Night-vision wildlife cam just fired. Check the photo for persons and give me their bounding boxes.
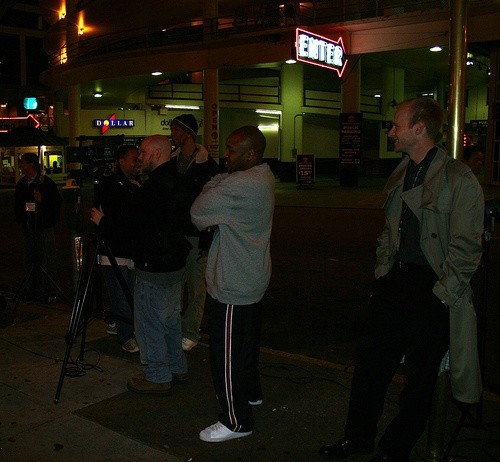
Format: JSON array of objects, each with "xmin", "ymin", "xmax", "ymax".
[
  {"xmin": 170, "ymin": 114, "xmax": 220, "ymax": 350},
  {"xmin": 90, "ymin": 135, "xmax": 189, "ymax": 393},
  {"xmin": 95, "ymin": 145, "xmax": 146, "ymax": 352},
  {"xmin": 189, "ymin": 126, "xmax": 276, "ymax": 443},
  {"xmin": 319, "ymin": 97, "xmax": 485, "ymax": 462},
  {"xmin": 14, "ymin": 152, "xmax": 60, "ymax": 304}
]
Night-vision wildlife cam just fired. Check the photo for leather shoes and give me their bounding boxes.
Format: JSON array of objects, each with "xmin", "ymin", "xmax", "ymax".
[
  {"xmin": 371, "ymin": 452, "xmax": 393, "ymax": 462},
  {"xmin": 321, "ymin": 434, "xmax": 374, "ymax": 457}
]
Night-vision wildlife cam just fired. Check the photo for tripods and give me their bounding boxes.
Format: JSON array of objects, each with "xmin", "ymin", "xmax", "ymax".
[
  {"xmin": 443, "ymin": 230, "xmax": 500, "ymax": 462},
  {"xmin": 8, "ymin": 176, "xmax": 76, "ymax": 326},
  {"xmin": 54, "ymin": 161, "xmax": 134, "ymax": 404}
]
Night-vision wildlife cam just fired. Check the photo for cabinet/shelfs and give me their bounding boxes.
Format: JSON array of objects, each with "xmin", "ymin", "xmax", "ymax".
[{"xmin": 64, "ymin": 134, "xmax": 173, "ymax": 181}]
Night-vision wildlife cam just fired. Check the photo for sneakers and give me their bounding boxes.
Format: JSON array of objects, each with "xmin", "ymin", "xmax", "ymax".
[
  {"xmin": 199, "ymin": 421, "xmax": 253, "ymax": 443},
  {"xmin": 172, "ymin": 373, "xmax": 188, "ymax": 381},
  {"xmin": 127, "ymin": 378, "xmax": 171, "ymax": 394}
]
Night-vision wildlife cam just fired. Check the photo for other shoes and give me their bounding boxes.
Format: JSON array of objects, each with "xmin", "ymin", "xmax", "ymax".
[
  {"xmin": 122, "ymin": 338, "xmax": 139, "ymax": 352},
  {"xmin": 181, "ymin": 337, "xmax": 197, "ymax": 350},
  {"xmin": 107, "ymin": 324, "xmax": 117, "ymax": 334}
]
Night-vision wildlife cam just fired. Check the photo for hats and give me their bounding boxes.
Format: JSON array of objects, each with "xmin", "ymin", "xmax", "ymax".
[{"xmin": 173, "ymin": 113, "xmax": 199, "ymax": 139}]
